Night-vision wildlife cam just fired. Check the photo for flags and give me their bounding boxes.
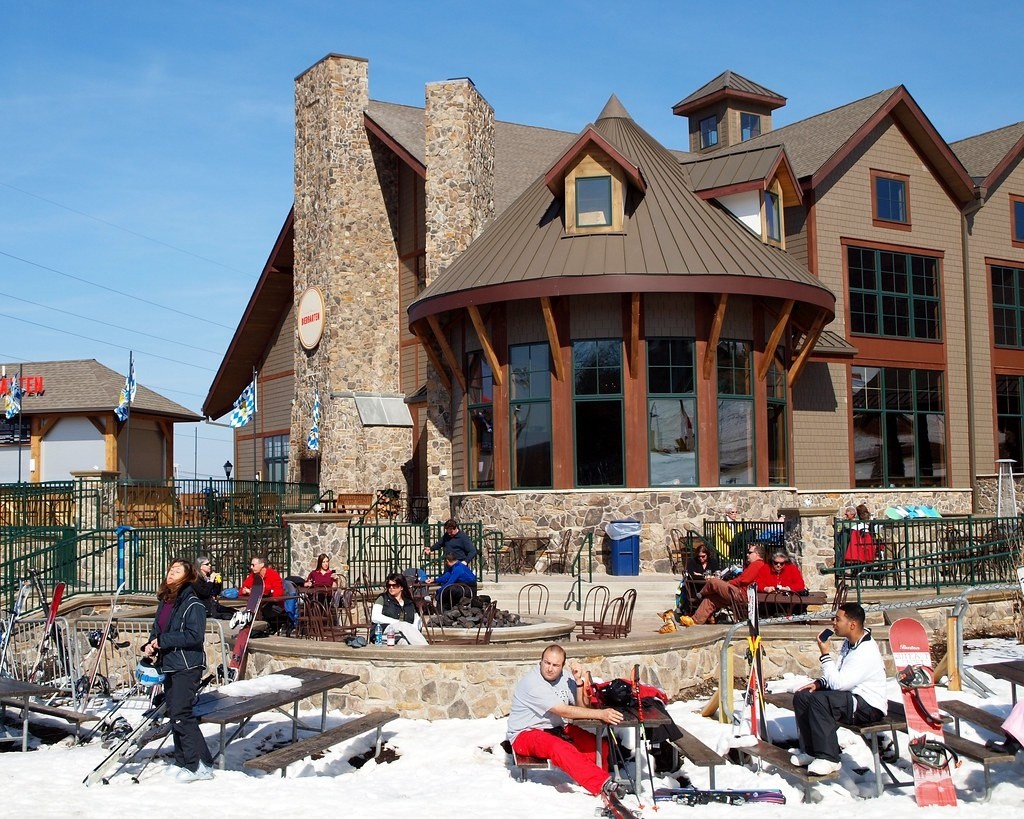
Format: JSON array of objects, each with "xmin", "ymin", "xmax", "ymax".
[
  {"xmin": 114, "ymin": 357, "xmax": 137, "ymax": 422},
  {"xmin": 306, "ymin": 389, "xmax": 322, "ymax": 452},
  {"xmin": 1, "ymin": 370, "xmax": 27, "ymax": 419},
  {"xmin": 228, "ymin": 380, "xmax": 257, "ymax": 429}
]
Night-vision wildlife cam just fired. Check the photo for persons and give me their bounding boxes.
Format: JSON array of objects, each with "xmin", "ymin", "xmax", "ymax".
[
  {"xmin": 238, "ymin": 556, "xmax": 286, "ymax": 635},
  {"xmin": 368, "ymin": 572, "xmax": 429, "ymax": 645},
  {"xmin": 191, "ymin": 554, "xmax": 233, "ymax": 620},
  {"xmin": 789, "ymin": 603, "xmax": 890, "ymax": 777},
  {"xmin": 141, "ymin": 557, "xmax": 216, "ymax": 782},
  {"xmin": 424, "ymin": 520, "xmax": 479, "ymax": 574},
  {"xmin": 425, "ymin": 552, "xmax": 478, "ymax": 615},
  {"xmin": 506, "ymin": 645, "xmax": 629, "ymax": 800},
  {"xmin": 303, "ymin": 554, "xmax": 340, "ymax": 614},
  {"xmin": 986, "ymin": 695, "xmax": 1023, "ymax": 756},
  {"xmin": 663, "ymin": 505, "xmax": 885, "ymax": 626}
]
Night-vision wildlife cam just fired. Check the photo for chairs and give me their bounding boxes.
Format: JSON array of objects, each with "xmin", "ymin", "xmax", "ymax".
[{"xmin": 212, "ymin": 517, "xmax": 1024, "ymax": 644}]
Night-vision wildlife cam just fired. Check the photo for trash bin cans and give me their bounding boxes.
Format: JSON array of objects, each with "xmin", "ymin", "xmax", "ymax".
[{"xmin": 604, "ymin": 519, "xmax": 642, "ymax": 576}]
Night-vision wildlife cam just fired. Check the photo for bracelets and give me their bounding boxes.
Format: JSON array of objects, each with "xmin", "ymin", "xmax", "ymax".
[{"xmin": 575, "ymin": 680, "xmax": 585, "ymax": 688}]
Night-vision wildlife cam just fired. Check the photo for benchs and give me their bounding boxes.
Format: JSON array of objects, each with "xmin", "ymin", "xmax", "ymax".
[
  {"xmin": 143, "ymin": 715, "xmax": 246, "ymax": 748},
  {"xmin": 330, "ymin": 494, "xmax": 373, "ymax": 526},
  {"xmin": 938, "ymin": 700, "xmax": 1007, "ymax": 739},
  {"xmin": 736, "ymin": 735, "xmax": 841, "ymax": 804},
  {"xmin": 511, "ymin": 745, "xmax": 549, "ymax": 782},
  {"xmin": 242, "ymin": 711, "xmax": 401, "ymax": 778},
  {"xmin": 0, "ymin": 508, "xmax": 167, "ymax": 527},
  {"xmin": 895, "ymin": 727, "xmax": 1015, "ymax": 802},
  {"xmin": 672, "ymin": 723, "xmax": 726, "ymax": 790},
  {"xmin": 0, "ymin": 696, "xmax": 102, "ymax": 747}
]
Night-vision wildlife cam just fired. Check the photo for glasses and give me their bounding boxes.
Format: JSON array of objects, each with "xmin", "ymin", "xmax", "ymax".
[
  {"xmin": 773, "ymin": 560, "xmax": 786, "ymax": 566},
  {"xmin": 250, "ymin": 563, "xmax": 262, "ymax": 567},
  {"xmin": 728, "ymin": 510, "xmax": 738, "ymax": 514},
  {"xmin": 386, "ymin": 583, "xmax": 404, "ymax": 589},
  {"xmin": 748, "ymin": 551, "xmax": 757, "ymax": 555},
  {"xmin": 201, "ymin": 562, "xmax": 211, "ymax": 567},
  {"xmin": 697, "ymin": 554, "xmax": 708, "ymax": 558}
]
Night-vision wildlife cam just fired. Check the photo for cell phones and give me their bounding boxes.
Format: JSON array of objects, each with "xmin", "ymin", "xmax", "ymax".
[
  {"xmin": 819, "ymin": 629, "xmax": 834, "ymax": 642},
  {"xmin": 332, "ymin": 570, "xmax": 336, "ymax": 573}
]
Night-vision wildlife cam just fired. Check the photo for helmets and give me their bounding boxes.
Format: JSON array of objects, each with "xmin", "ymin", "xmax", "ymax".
[{"xmin": 135, "ymin": 658, "xmax": 168, "ymax": 689}]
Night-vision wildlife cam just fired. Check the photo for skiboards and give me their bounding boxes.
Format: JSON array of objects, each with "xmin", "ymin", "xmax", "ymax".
[
  {"xmin": 18, "ymin": 581, "xmax": 67, "ymax": 719},
  {"xmin": 747, "ymin": 581, "xmax": 771, "ymax": 742},
  {"xmin": 0, "ymin": 584, "xmax": 28, "ymax": 677},
  {"xmin": 80, "ymin": 582, "xmax": 126, "ymax": 714}
]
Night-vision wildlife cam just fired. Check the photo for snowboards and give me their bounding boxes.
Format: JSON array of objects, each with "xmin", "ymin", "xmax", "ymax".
[
  {"xmin": 226, "ymin": 572, "xmax": 264, "ymax": 686},
  {"xmin": 651, "ymin": 787, "xmax": 787, "ymax": 806},
  {"xmin": 601, "ymin": 790, "xmax": 645, "ymax": 819},
  {"xmin": 888, "ymin": 618, "xmax": 962, "ymax": 807}
]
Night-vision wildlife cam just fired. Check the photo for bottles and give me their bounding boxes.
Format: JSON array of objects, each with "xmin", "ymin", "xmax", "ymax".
[
  {"xmin": 703, "ymin": 568, "xmax": 721, "ymax": 580},
  {"xmin": 387, "ymin": 625, "xmax": 395, "ymax": 646},
  {"xmin": 374, "ymin": 621, "xmax": 382, "ymax": 647}
]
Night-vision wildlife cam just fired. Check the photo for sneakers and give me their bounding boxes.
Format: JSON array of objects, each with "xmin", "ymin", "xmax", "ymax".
[
  {"xmin": 679, "ymin": 614, "xmax": 698, "ymax": 627},
  {"xmin": 607, "ymin": 780, "xmax": 629, "ymax": 800},
  {"xmin": 807, "ymin": 758, "xmax": 842, "ymax": 775},
  {"xmin": 790, "ymin": 751, "xmax": 817, "ymax": 767},
  {"xmin": 169, "ymin": 764, "xmax": 189, "ymax": 776},
  {"xmin": 176, "ymin": 756, "xmax": 214, "ymax": 782}
]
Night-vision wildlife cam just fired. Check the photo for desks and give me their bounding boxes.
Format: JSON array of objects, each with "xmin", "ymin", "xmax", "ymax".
[
  {"xmin": 764, "ymin": 690, "xmax": 954, "ymax": 798},
  {"xmin": 168, "ymin": 667, "xmax": 360, "ymax": 772},
  {"xmin": 0, "ymin": 678, "xmax": 61, "ymax": 754},
  {"xmin": 490, "ymin": 536, "xmax": 552, "ymax": 575},
  {"xmin": 573, "ymin": 705, "xmax": 671, "ymax": 797},
  {"xmin": 974, "ymin": 660, "xmax": 1024, "ymax": 708},
  {"xmin": 314, "ymin": 499, "xmax": 339, "ymax": 513}
]
